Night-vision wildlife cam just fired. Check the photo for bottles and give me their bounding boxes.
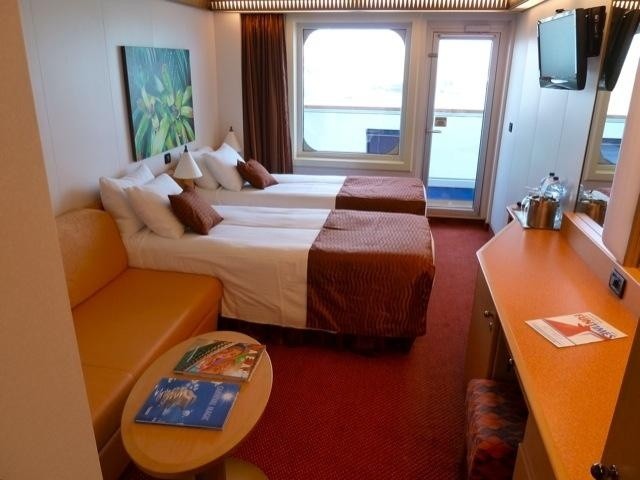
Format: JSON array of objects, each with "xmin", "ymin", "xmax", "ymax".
[
  {"xmin": 543, "ymin": 177, "xmax": 564, "ymax": 230},
  {"xmin": 538, "ymin": 172, "xmax": 556, "ymax": 199}
]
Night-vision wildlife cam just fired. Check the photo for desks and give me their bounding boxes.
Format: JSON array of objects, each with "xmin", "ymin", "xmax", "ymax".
[{"xmin": 467, "ymin": 203, "xmax": 637, "ymax": 477}]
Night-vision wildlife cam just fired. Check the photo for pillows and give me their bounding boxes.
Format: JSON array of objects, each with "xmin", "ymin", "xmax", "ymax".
[
  {"xmin": 203, "ymin": 142, "xmax": 246, "ymax": 192},
  {"xmin": 100, "ymin": 163, "xmax": 155, "ymax": 240},
  {"xmin": 236, "ymin": 159, "xmax": 279, "ymax": 189},
  {"xmin": 167, "ymin": 186, "xmax": 224, "ymax": 235},
  {"xmin": 123, "ymin": 173, "xmax": 186, "ymax": 241},
  {"xmin": 176, "ymin": 146, "xmax": 220, "ymax": 190}
]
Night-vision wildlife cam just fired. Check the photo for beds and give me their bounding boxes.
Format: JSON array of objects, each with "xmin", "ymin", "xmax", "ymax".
[
  {"xmin": 99, "ymin": 204, "xmax": 433, "ymax": 352},
  {"xmin": 173, "ymin": 167, "xmax": 428, "ymax": 217}
]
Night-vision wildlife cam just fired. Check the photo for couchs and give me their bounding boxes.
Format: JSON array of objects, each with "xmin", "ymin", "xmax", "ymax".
[{"xmin": 57, "ymin": 207, "xmax": 226, "ymax": 479}]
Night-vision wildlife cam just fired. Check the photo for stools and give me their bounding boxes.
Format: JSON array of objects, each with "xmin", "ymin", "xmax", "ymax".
[{"xmin": 466, "ymin": 376, "xmax": 530, "ymax": 479}]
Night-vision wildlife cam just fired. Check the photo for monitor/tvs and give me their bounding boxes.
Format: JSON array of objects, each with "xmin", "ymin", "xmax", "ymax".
[
  {"xmin": 605, "ymin": 9, "xmax": 640, "ymax": 91},
  {"xmin": 537, "ymin": 7, "xmax": 587, "ymax": 90}
]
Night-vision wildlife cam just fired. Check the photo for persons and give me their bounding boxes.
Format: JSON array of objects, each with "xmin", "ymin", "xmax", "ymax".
[{"xmin": 188, "ymin": 344, "xmax": 245, "ymax": 375}]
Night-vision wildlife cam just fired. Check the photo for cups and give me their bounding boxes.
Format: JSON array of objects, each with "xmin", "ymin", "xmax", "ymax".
[
  {"xmin": 527, "ymin": 193, "xmax": 557, "ymax": 229},
  {"xmin": 576, "ymin": 197, "xmax": 605, "ymax": 224}
]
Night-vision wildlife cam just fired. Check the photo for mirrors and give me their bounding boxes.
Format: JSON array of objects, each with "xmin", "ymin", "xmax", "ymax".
[{"xmin": 575, "ymin": 0, "xmax": 640, "ymax": 240}]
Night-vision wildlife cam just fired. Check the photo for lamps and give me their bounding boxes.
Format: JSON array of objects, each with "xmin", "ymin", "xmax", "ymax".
[
  {"xmin": 225, "ymin": 126, "xmax": 242, "ymax": 152},
  {"xmin": 173, "ymin": 144, "xmax": 203, "ymax": 189}
]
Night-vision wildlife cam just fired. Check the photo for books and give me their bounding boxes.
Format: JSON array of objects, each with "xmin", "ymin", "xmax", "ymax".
[
  {"xmin": 171, "ymin": 336, "xmax": 268, "ymax": 382},
  {"xmin": 134, "ymin": 375, "xmax": 240, "ymax": 430}
]
